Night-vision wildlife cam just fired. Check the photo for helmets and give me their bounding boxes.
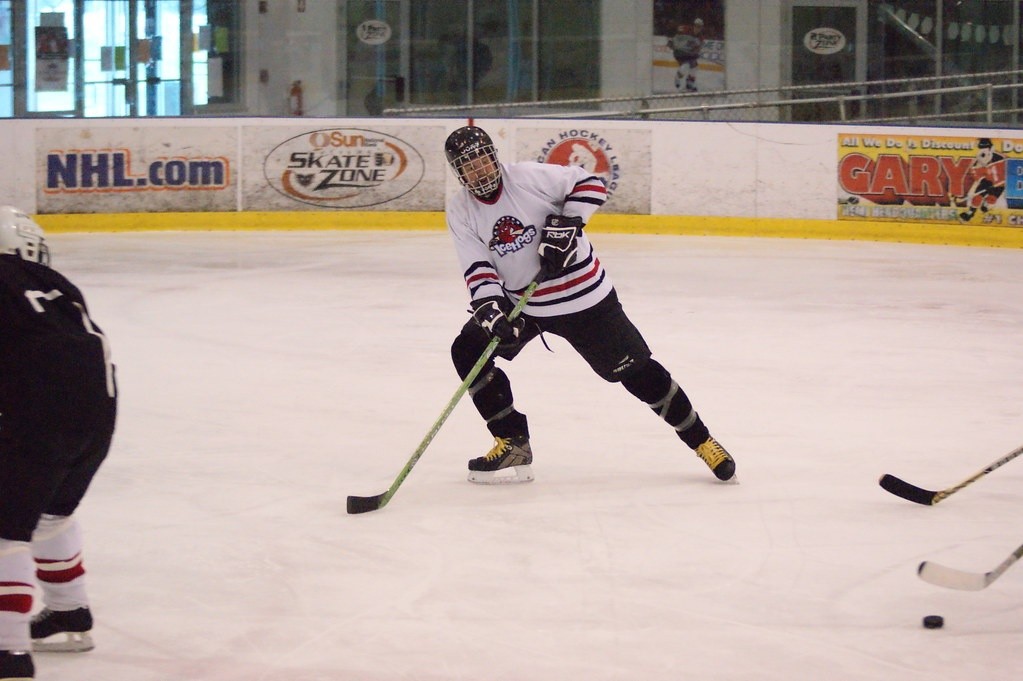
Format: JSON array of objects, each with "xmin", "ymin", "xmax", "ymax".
[
  {"xmin": 444, "ymin": 126, "xmax": 500, "ymax": 200},
  {"xmin": 0, "ymin": 205, "xmax": 50, "ymax": 266}
]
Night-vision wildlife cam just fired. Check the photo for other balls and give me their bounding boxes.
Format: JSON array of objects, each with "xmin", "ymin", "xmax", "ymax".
[{"xmin": 923, "ymin": 615, "xmax": 943, "ymax": 629}]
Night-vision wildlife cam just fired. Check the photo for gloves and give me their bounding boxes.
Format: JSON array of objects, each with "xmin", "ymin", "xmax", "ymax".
[
  {"xmin": 471, "ymin": 296, "xmax": 526, "ymax": 348},
  {"xmin": 538, "ymin": 215, "xmax": 585, "ymax": 278}
]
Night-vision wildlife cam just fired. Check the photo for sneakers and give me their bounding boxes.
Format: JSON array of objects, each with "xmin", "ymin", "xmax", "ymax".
[
  {"xmin": 0, "ymin": 647, "xmax": 35, "ymax": 681},
  {"xmin": 469, "ymin": 432, "xmax": 535, "ymax": 483},
  {"xmin": 693, "ymin": 436, "xmax": 741, "ymax": 484},
  {"xmin": 31, "ymin": 606, "xmax": 94, "ymax": 653}
]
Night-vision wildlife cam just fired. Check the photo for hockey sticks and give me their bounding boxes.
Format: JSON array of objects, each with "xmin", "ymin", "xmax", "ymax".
[
  {"xmin": 915, "ymin": 540, "xmax": 1023, "ymax": 595},
  {"xmin": 346, "ymin": 258, "xmax": 544, "ymax": 517},
  {"xmin": 877, "ymin": 441, "xmax": 1023, "ymax": 507}
]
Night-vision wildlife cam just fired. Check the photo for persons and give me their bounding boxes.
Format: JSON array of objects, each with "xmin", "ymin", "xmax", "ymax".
[
  {"xmin": 444, "ymin": 126, "xmax": 738, "ymax": 485},
  {"xmin": 667, "ymin": 18, "xmax": 705, "ymax": 93},
  {"xmin": 0, "ymin": 205, "xmax": 118, "ymax": 681}
]
